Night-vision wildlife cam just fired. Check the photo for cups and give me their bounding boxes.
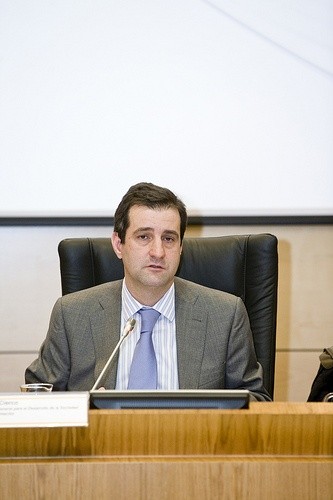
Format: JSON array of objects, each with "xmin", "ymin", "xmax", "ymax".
[{"xmin": 20, "ymin": 383, "xmax": 53, "ymax": 392}]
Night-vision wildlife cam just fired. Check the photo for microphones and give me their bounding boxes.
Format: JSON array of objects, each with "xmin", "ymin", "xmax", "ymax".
[{"xmin": 91, "ymin": 318, "xmax": 136, "ymax": 391}]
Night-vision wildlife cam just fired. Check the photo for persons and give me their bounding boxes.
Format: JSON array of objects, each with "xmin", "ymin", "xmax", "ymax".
[{"xmin": 23, "ymin": 181, "xmax": 273, "ymax": 402}]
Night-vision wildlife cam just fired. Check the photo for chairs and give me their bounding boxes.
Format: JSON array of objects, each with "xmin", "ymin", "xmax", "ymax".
[{"xmin": 58, "ymin": 233, "xmax": 280, "ymax": 401}]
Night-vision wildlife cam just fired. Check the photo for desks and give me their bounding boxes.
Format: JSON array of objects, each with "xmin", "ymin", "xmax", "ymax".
[{"xmin": 0, "ymin": 400, "xmax": 333, "ymax": 500}]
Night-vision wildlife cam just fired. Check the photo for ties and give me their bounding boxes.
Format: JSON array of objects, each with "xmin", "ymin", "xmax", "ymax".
[{"xmin": 126, "ymin": 307, "xmax": 161, "ymax": 389}]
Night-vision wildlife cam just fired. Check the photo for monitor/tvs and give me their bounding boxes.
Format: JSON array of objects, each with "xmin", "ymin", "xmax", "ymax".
[{"xmin": 89, "ymin": 389, "xmax": 250, "ymax": 410}]
